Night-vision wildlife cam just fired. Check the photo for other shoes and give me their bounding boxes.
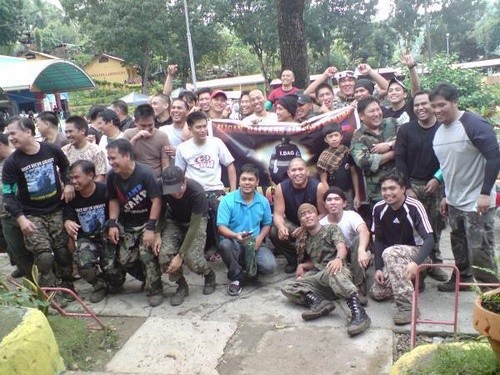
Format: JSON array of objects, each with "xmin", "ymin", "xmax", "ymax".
[
  {"xmin": 346, "ymin": 295, "xmax": 372, "ymax": 336},
  {"xmin": 357, "ymin": 291, "xmax": 369, "ymax": 304},
  {"xmin": 89, "ymin": 284, "xmax": 108, "ymax": 303},
  {"xmin": 203, "ymin": 271, "xmax": 216, "ymax": 295},
  {"xmin": 301, "ymin": 291, "xmax": 336, "ymax": 321},
  {"xmin": 10, "ymin": 267, "xmax": 26, "ymax": 279},
  {"xmin": 431, "ymin": 266, "xmax": 449, "ymax": 280},
  {"xmin": 227, "ymin": 282, "xmax": 242, "ymax": 296},
  {"xmin": 393, "ymin": 309, "xmax": 421, "ymax": 324},
  {"xmin": 61, "ymin": 279, "xmax": 77, "ymax": 302},
  {"xmin": 46, "ymin": 289, "xmax": 68, "ymax": 308},
  {"xmin": 285, "ymin": 263, "xmax": 298, "ymax": 274},
  {"xmin": 149, "ymin": 292, "xmax": 163, "ymax": 306},
  {"xmin": 413, "ymin": 273, "xmax": 425, "ymax": 292},
  {"xmin": 170, "ymin": 281, "xmax": 189, "ymax": 306},
  {"xmin": 437, "ymin": 274, "xmax": 475, "ymax": 293}
]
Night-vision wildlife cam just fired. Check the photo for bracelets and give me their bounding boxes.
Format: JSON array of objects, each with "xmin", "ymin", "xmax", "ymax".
[
  {"xmin": 105, "ymin": 218, "xmax": 118, "ymax": 227},
  {"xmin": 336, "ymin": 256, "xmax": 344, "ymax": 261},
  {"xmin": 146, "ymin": 218, "xmax": 157, "ymax": 231},
  {"xmin": 168, "ymin": 73, "xmax": 174, "ymax": 76},
  {"xmin": 408, "ymin": 63, "xmax": 417, "ymax": 69}
]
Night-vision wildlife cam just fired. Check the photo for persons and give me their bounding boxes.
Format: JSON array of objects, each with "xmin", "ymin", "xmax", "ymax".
[
  {"xmin": 216, "ymin": 163, "xmax": 276, "ymax": 295},
  {"xmin": 268, "ymin": 70, "xmax": 302, "ymax": 103},
  {"xmin": 105, "ymin": 138, "xmax": 163, "ymax": 306},
  {"xmin": 280, "ymin": 203, "xmax": 371, "ymax": 336},
  {"xmin": 375, "ymin": 54, "xmax": 420, "ymax": 133},
  {"xmin": 62, "ymin": 159, "xmax": 126, "ymax": 303},
  {"xmin": 163, "ymin": 64, "xmax": 179, "ymax": 103},
  {"xmin": 151, "ymin": 166, "xmax": 215, "ymax": 306},
  {"xmin": 369, "ymin": 176, "xmax": 435, "ymax": 324},
  {"xmin": 0, "ymin": 78, "xmax": 448, "ymax": 293},
  {"xmin": 429, "ymin": 83, "xmax": 500, "ymax": 292},
  {"xmin": 1, "ymin": 117, "xmax": 76, "ymax": 300},
  {"xmin": 303, "ymin": 64, "xmax": 390, "ymax": 97},
  {"xmin": 270, "ymin": 157, "xmax": 327, "ymax": 273},
  {"xmin": 317, "ymin": 189, "xmax": 371, "ymax": 269}
]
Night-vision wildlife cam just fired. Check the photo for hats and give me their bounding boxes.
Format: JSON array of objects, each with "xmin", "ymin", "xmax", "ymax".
[
  {"xmin": 386, "ymin": 77, "xmax": 408, "ymax": 91},
  {"xmin": 163, "ymin": 165, "xmax": 186, "ymax": 195},
  {"xmin": 296, "ymin": 94, "xmax": 313, "ymax": 104},
  {"xmin": 353, "ymin": 79, "xmax": 376, "ymax": 95},
  {"xmin": 278, "ymin": 94, "xmax": 299, "ymax": 118},
  {"xmin": 209, "ymin": 89, "xmax": 227, "ymax": 100},
  {"xmin": 323, "ymin": 186, "xmax": 347, "ymax": 201}
]
